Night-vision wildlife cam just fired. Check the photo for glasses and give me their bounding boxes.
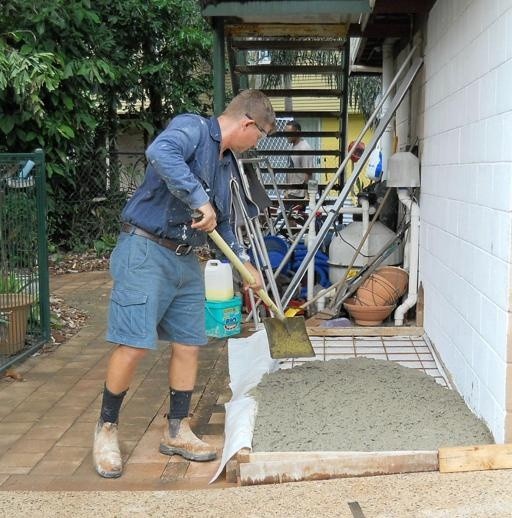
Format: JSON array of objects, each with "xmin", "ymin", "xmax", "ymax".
[{"xmin": 245, "ymin": 114, "xmax": 269, "ymax": 140}]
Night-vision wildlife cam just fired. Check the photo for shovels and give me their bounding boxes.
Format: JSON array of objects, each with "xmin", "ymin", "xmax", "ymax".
[{"xmin": 191, "ymin": 211, "xmax": 315, "ymax": 358}]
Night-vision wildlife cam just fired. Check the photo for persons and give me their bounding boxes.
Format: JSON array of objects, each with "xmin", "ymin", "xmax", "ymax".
[
  {"xmin": 283, "ymin": 121, "xmax": 316, "ymax": 226},
  {"xmin": 91, "ymin": 87, "xmax": 278, "ymax": 479}
]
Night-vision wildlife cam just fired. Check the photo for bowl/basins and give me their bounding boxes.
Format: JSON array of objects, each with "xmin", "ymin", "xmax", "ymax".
[
  {"xmin": 343, "ymin": 297, "xmax": 397, "ymax": 326},
  {"xmin": 354, "ymin": 265, "xmax": 408, "ymax": 308}
]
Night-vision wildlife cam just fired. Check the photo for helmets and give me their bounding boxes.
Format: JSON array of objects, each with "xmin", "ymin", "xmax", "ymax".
[{"xmin": 348, "ymin": 140, "xmax": 365, "ymax": 162}]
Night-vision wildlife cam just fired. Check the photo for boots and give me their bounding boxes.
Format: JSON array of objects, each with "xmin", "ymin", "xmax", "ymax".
[
  {"xmin": 159, "ymin": 413, "xmax": 217, "ymax": 462},
  {"xmin": 93, "ymin": 418, "xmax": 123, "ymax": 478}
]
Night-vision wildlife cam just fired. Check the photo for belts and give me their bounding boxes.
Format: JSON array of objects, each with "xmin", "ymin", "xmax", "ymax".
[{"xmin": 121, "ymin": 223, "xmax": 193, "ymax": 255}]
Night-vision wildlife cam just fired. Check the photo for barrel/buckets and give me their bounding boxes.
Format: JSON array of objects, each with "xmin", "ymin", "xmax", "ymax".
[
  {"xmin": 250, "ymin": 236, "xmax": 288, "ymax": 271},
  {"xmin": 203, "ymin": 258, "xmax": 234, "ymax": 301},
  {"xmin": 204, "ymin": 297, "xmax": 242, "ymax": 339}
]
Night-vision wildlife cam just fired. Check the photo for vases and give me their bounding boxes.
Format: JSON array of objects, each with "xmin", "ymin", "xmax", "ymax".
[
  {"xmin": 342, "ymin": 267, "xmax": 410, "ymax": 327},
  {"xmin": 0, "ymin": 294, "xmax": 36, "ymax": 354}
]
